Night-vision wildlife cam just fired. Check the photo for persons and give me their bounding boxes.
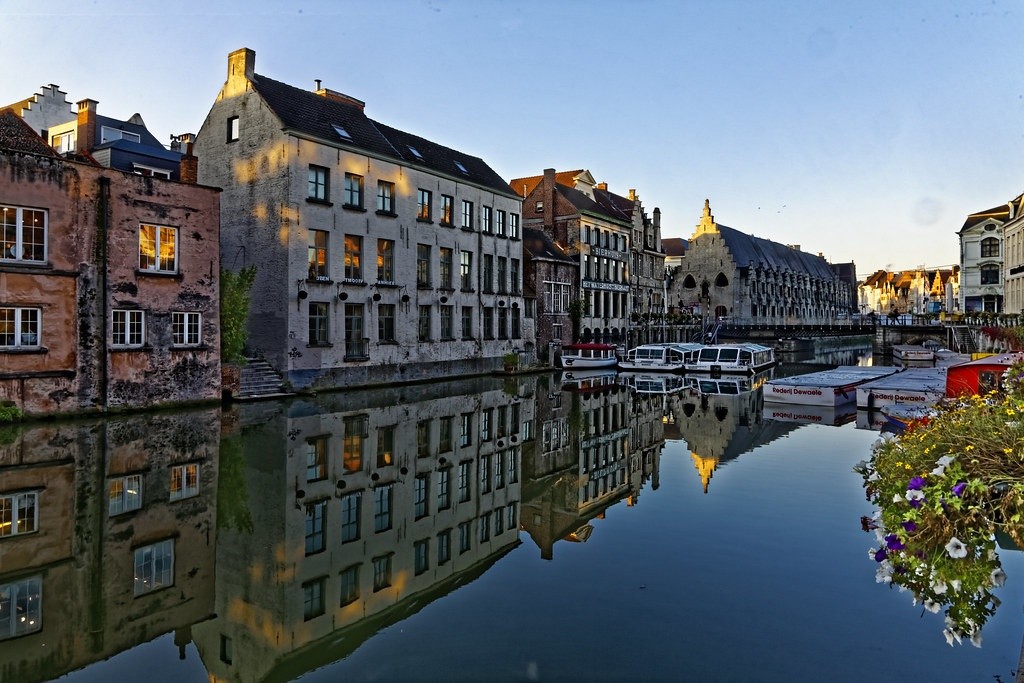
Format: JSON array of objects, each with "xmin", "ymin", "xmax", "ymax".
[
  {"xmin": 888, "ymin": 304, "xmax": 900, "ymax": 325},
  {"xmin": 869, "ymin": 310, "xmax": 876, "ymax": 325}
]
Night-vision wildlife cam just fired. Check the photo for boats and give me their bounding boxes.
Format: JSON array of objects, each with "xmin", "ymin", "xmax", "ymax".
[
  {"xmin": 618, "ymin": 342, "xmax": 700, "ymax": 370},
  {"xmin": 558, "ymin": 368, "xmax": 618, "ymax": 393},
  {"xmin": 878, "ymin": 350, "xmax": 1024, "ymax": 431},
  {"xmin": 685, "ymin": 342, "xmax": 777, "ymax": 371},
  {"xmin": 618, "ymin": 372, "xmax": 692, "ymax": 395},
  {"xmin": 683, "ymin": 366, "xmax": 775, "ymax": 397},
  {"xmin": 560, "ymin": 343, "xmax": 617, "ymax": 368}
]
